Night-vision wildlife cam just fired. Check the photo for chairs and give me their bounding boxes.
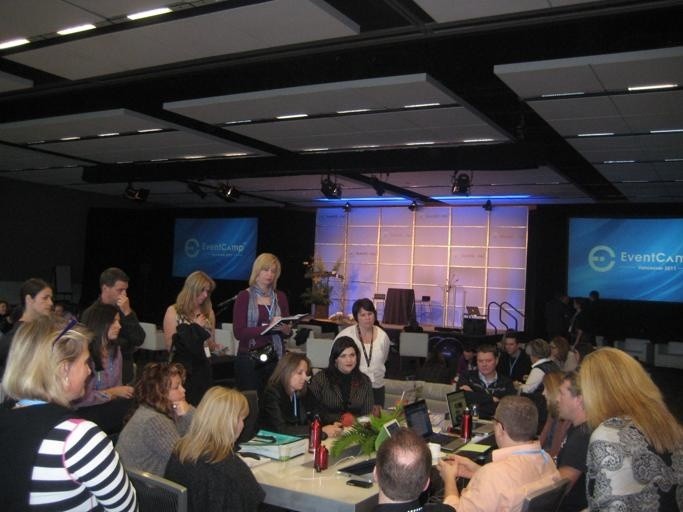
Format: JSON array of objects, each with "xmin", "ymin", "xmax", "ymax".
[
  {"xmin": 306, "ymin": 332, "xmax": 333, "ymax": 370},
  {"xmin": 614, "ymin": 339, "xmax": 650, "ymax": 364},
  {"xmin": 123, "ymin": 468, "xmax": 188, "ymax": 512},
  {"xmin": 521, "ymin": 479, "xmax": 575, "ymax": 512},
  {"xmin": 213, "ymin": 329, "xmax": 233, "ymax": 356},
  {"xmin": 398, "ymin": 331, "xmax": 429, "ymax": 378},
  {"xmin": 654, "ymin": 342, "xmax": 683, "ymax": 368},
  {"xmin": 138, "ymin": 322, "xmax": 170, "ymax": 351}
]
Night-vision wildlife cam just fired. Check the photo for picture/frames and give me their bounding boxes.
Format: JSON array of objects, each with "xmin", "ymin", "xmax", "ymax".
[
  {"xmin": 384, "ymin": 419, "xmax": 402, "ymax": 437},
  {"xmin": 447, "ymin": 390, "xmax": 471, "ymax": 426}
]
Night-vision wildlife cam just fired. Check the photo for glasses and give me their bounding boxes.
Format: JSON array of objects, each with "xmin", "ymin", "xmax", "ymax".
[
  {"xmin": 491, "ymin": 417, "xmax": 504, "ymax": 430},
  {"xmin": 51, "ymin": 315, "xmax": 76, "ymax": 353}
]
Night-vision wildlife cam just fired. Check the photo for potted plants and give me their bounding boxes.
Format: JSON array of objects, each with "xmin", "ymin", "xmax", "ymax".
[{"xmin": 299, "ymin": 253, "xmax": 344, "ymax": 319}]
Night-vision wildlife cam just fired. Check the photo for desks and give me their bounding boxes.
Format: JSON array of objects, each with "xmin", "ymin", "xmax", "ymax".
[
  {"xmin": 233, "ymin": 404, "xmax": 499, "ymax": 512},
  {"xmin": 298, "ymin": 317, "xmax": 524, "ymax": 342}
]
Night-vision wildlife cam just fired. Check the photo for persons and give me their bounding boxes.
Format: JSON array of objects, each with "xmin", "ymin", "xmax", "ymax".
[
  {"xmin": 550, "ymin": 337, "xmax": 568, "ymax": 366},
  {"xmin": 55, "ymin": 304, "xmax": 64, "ymax": 316},
  {"xmin": 539, "ymin": 371, "xmax": 572, "ymax": 461},
  {"xmin": 309, "ymin": 336, "xmax": 374, "ymax": 415},
  {"xmin": 81, "ymin": 268, "xmax": 145, "ymax": 385},
  {"xmin": 556, "ymin": 373, "xmax": 596, "ymax": 512},
  {"xmin": 576, "ymin": 348, "xmax": 683, "ymax": 512},
  {"xmin": 115, "ymin": 362, "xmax": 196, "ymax": 478},
  {"xmin": 454, "ymin": 345, "xmax": 478, "ymax": 382},
  {"xmin": 513, "ymin": 340, "xmax": 560, "ymax": 436},
  {"xmin": 569, "ymin": 298, "xmax": 592, "ymax": 357},
  {"xmin": 0, "ymin": 301, "xmax": 13, "ymax": 333},
  {"xmin": 437, "ymin": 396, "xmax": 558, "ymax": 512},
  {"xmin": 234, "ymin": 254, "xmax": 293, "ymax": 392},
  {"xmin": 405, "ymin": 318, "xmax": 423, "ymax": 333},
  {"xmin": 261, "ymin": 352, "xmax": 345, "ymax": 438},
  {"xmin": 69, "ymin": 304, "xmax": 135, "ymax": 446},
  {"xmin": 329, "ymin": 298, "xmax": 390, "ymax": 409},
  {"xmin": 457, "ymin": 344, "xmax": 517, "ymax": 419},
  {"xmin": 164, "ymin": 386, "xmax": 265, "ymax": 512},
  {"xmin": 0, "ymin": 279, "xmax": 53, "ymax": 404},
  {"xmin": 590, "ymin": 290, "xmax": 601, "ymax": 303},
  {"xmin": 370, "ymin": 428, "xmax": 456, "ymax": 512},
  {"xmin": 164, "ymin": 271, "xmax": 216, "ymax": 409},
  {"xmin": 0, "ymin": 315, "xmax": 139, "ymax": 512},
  {"xmin": 497, "ymin": 333, "xmax": 532, "ymax": 383},
  {"xmin": 547, "ymin": 295, "xmax": 577, "ymax": 342}
]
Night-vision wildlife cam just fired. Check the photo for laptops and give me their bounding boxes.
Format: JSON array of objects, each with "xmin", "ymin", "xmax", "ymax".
[
  {"xmin": 404, "ymin": 399, "xmax": 469, "ymax": 452},
  {"xmin": 447, "ymin": 390, "xmax": 495, "ymax": 436}
]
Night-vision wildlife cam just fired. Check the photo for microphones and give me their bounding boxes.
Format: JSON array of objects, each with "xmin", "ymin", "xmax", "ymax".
[{"xmin": 218, "ymin": 294, "xmax": 237, "ymax": 307}]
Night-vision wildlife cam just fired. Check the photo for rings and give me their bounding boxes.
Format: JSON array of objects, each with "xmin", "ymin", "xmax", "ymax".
[{"xmin": 173, "ymin": 404, "xmax": 177, "ymax": 409}]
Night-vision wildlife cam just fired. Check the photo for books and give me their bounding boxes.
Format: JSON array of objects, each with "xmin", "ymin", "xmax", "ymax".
[{"xmin": 260, "ymin": 313, "xmax": 309, "ymax": 336}]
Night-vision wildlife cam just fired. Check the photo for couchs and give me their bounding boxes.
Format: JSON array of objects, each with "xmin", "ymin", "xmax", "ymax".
[{"xmin": 382, "ymin": 379, "xmax": 454, "ymax": 414}]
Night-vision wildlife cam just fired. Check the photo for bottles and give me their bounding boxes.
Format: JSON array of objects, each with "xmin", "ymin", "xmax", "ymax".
[
  {"xmin": 461, "ymin": 407, "xmax": 472, "ymax": 438},
  {"xmin": 308, "ymin": 414, "xmax": 322, "ymax": 453},
  {"xmin": 314, "ymin": 446, "xmax": 329, "ymax": 471}
]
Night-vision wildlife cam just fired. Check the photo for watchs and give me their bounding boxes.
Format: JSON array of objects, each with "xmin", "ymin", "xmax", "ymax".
[{"xmin": 518, "ymin": 382, "xmax": 521, "ymax": 386}]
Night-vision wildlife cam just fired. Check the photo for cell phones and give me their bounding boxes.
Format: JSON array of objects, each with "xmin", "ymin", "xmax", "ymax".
[{"xmin": 348, "ymin": 478, "xmax": 373, "ymax": 488}]
